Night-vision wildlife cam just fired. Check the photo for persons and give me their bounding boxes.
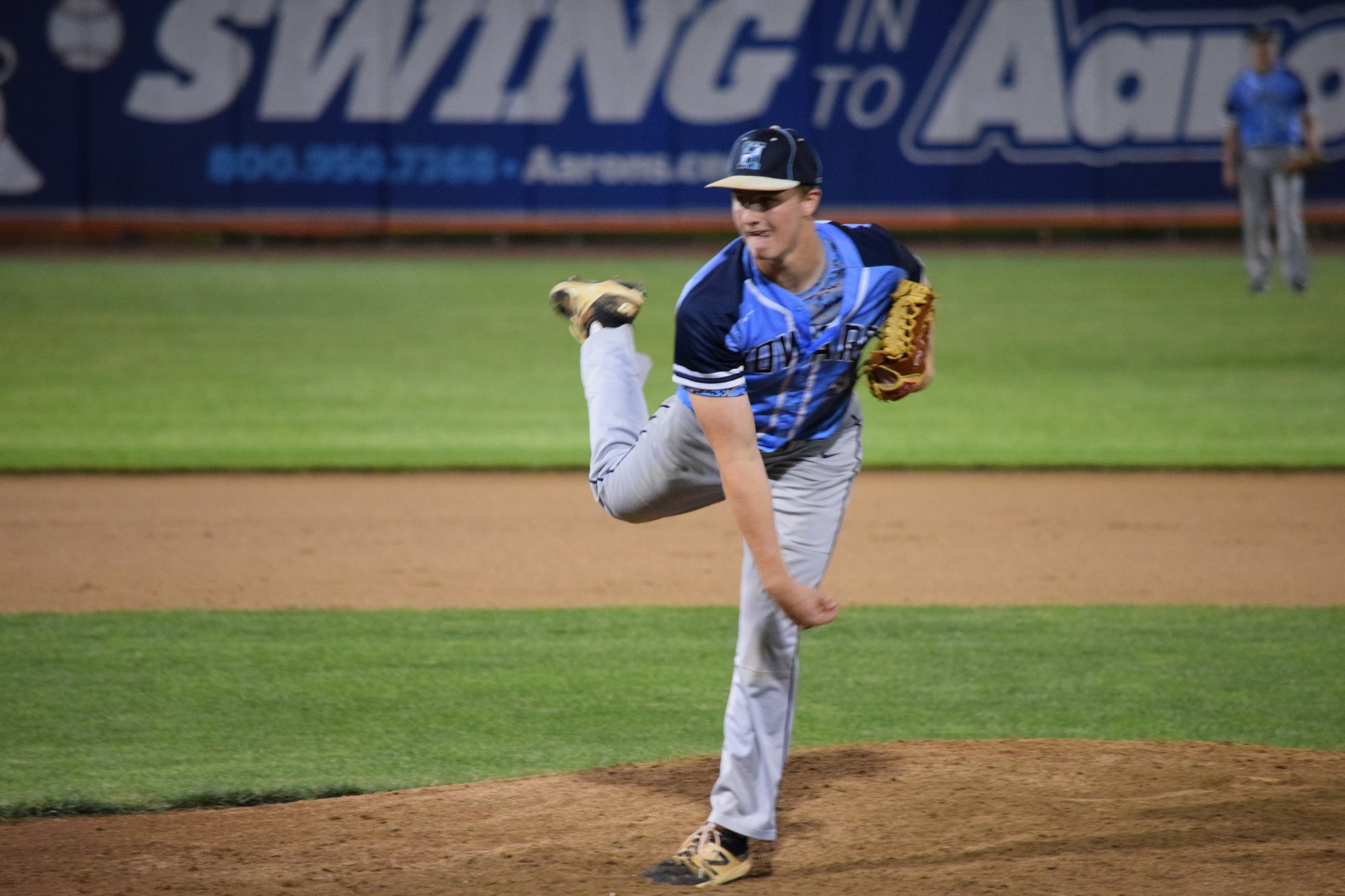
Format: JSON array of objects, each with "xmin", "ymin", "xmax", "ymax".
[
  {"xmin": 551, "ymin": 125, "xmax": 936, "ymax": 888},
  {"xmin": 1218, "ymin": 25, "xmax": 1317, "ymax": 296}
]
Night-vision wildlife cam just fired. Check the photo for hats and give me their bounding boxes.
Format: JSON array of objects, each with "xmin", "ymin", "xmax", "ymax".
[{"xmin": 704, "ymin": 125, "xmax": 823, "ymax": 190}]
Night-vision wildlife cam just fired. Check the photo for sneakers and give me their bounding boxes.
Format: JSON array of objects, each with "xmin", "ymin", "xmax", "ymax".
[
  {"xmin": 641, "ymin": 824, "xmax": 754, "ymax": 889},
  {"xmin": 549, "ymin": 279, "xmax": 646, "ymax": 342}
]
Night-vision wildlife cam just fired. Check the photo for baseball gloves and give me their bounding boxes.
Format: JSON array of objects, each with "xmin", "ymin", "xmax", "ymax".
[
  {"xmin": 866, "ymin": 280, "xmax": 937, "ymax": 403},
  {"xmin": 1278, "ymin": 154, "xmax": 1318, "ymax": 181}
]
